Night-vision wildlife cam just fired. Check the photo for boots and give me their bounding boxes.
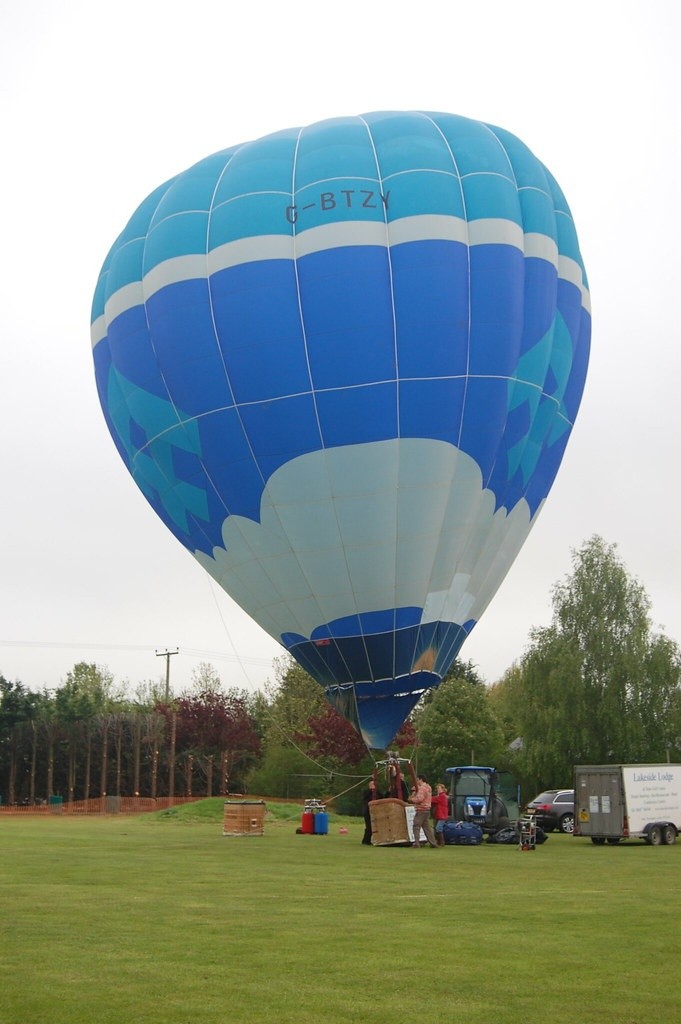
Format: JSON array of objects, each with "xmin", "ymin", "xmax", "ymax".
[{"xmin": 436, "ymin": 832, "xmax": 445, "ymax": 847}]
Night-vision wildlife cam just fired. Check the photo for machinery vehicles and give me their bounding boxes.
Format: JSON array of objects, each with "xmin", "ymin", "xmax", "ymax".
[{"xmin": 443, "ymin": 766, "xmax": 510, "ymax": 838}]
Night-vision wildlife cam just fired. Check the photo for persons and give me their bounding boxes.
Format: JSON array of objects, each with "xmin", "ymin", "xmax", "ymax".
[
  {"xmin": 362, "ymin": 773, "xmax": 448, "ymax": 846},
  {"xmin": 408, "ymin": 775, "xmax": 437, "ymax": 847}
]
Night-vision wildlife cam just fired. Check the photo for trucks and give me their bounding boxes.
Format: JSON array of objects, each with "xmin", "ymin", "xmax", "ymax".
[{"xmin": 571, "ymin": 763, "xmax": 681, "ymax": 845}]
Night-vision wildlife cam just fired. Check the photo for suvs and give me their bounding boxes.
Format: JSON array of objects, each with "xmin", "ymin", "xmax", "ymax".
[{"xmin": 525, "ymin": 789, "xmax": 575, "ymax": 834}]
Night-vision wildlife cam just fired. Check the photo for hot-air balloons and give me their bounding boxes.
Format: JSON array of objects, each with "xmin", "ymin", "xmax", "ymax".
[{"xmin": 86, "ymin": 107, "xmax": 591, "ymax": 847}]
